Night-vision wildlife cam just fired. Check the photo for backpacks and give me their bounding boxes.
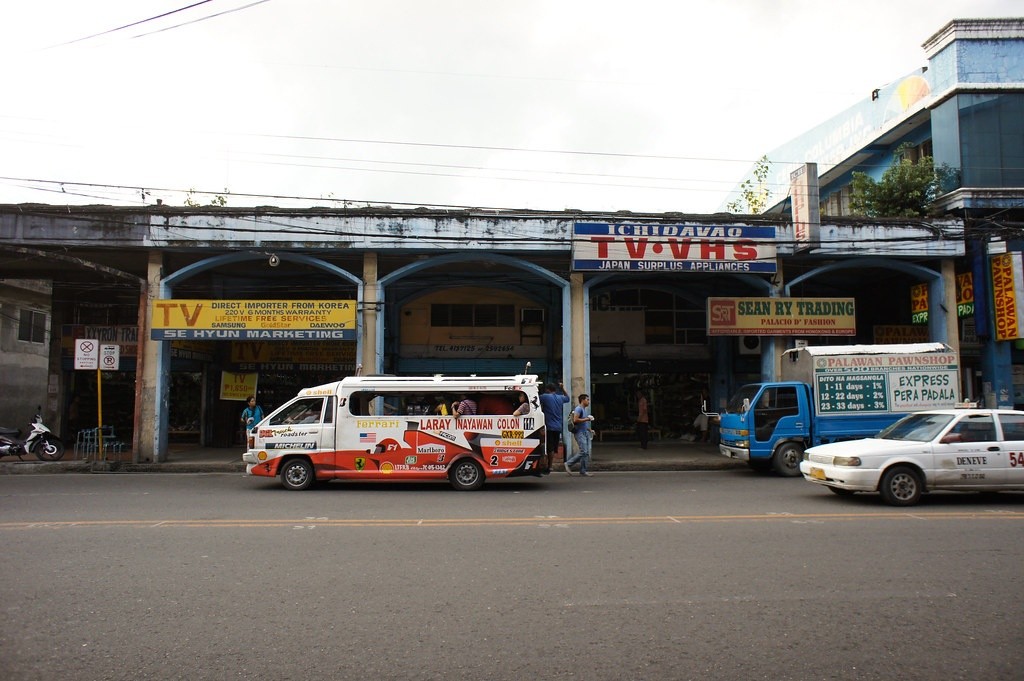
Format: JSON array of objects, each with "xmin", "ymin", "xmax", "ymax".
[{"xmin": 568, "ymin": 406, "xmax": 583, "ymax": 434}]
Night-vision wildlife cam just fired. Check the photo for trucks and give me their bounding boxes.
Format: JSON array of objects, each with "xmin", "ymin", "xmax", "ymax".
[{"xmin": 702, "ymin": 342, "xmax": 962, "ymax": 478}]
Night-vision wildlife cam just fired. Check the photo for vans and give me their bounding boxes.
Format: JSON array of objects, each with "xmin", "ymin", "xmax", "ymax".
[{"xmin": 242, "ymin": 362, "xmax": 549, "ymax": 491}]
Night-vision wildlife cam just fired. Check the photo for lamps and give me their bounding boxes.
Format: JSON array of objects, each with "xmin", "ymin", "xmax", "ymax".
[{"xmin": 268, "ymin": 252, "xmax": 280, "ymax": 266}]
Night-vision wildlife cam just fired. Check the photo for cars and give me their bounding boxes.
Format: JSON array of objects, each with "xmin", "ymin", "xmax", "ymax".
[{"xmin": 799, "ymin": 400, "xmax": 1024, "ymax": 506}]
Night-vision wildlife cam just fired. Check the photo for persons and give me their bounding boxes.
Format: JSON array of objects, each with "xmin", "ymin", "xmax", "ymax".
[
  {"xmin": 242, "ymin": 396, "xmax": 265, "ymax": 434},
  {"xmin": 539, "ymin": 382, "xmax": 570, "ymax": 477},
  {"xmin": 636, "ymin": 388, "xmax": 649, "ymax": 450},
  {"xmin": 564, "ymin": 393, "xmax": 596, "ymax": 477},
  {"xmin": 423, "ymin": 392, "xmax": 529, "ymax": 417},
  {"xmin": 284, "ymin": 404, "xmax": 325, "ymax": 424}
]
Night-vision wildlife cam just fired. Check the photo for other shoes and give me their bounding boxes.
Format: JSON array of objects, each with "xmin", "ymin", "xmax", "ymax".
[
  {"xmin": 564, "ymin": 462, "xmax": 573, "ymax": 477},
  {"xmin": 582, "ymin": 472, "xmax": 594, "ymax": 477}
]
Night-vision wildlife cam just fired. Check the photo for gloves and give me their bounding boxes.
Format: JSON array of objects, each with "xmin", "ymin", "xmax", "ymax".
[{"xmin": 588, "ymin": 415, "xmax": 595, "ymax": 421}]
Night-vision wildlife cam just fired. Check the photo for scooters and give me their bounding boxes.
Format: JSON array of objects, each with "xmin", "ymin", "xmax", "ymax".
[{"xmin": 0, "ymin": 404, "xmax": 65, "ymax": 461}]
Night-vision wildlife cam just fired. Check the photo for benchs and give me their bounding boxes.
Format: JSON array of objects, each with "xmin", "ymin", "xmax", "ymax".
[{"xmin": 597, "ymin": 428, "xmax": 661, "ymax": 441}]
